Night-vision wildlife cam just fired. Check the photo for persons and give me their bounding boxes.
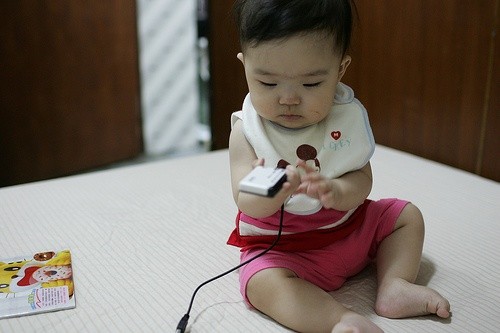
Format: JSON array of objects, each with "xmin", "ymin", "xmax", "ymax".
[{"xmin": 226, "ymin": 0, "xmax": 450, "ymax": 333}]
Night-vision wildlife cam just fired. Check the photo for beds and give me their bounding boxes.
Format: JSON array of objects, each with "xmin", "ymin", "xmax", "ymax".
[{"xmin": 0, "ymin": 143, "xmax": 500, "ymax": 333}]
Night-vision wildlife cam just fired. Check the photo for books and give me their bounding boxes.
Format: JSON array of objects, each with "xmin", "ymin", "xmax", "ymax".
[{"xmin": 0, "ymin": 249, "xmax": 76, "ymax": 319}]
